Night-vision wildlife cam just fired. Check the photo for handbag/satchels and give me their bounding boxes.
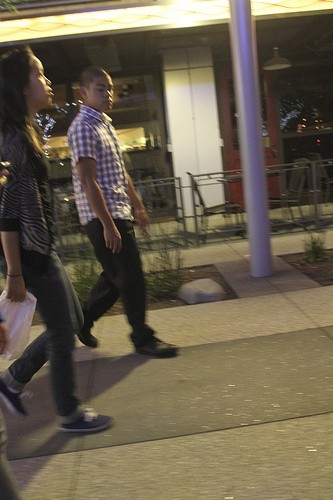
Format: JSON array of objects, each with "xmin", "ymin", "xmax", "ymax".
[{"xmin": 0, "ymin": 286, "xmax": 36, "ymax": 360}]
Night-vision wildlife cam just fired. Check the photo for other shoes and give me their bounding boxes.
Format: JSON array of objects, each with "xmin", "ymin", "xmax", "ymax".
[
  {"xmin": 56, "ymin": 406, "xmax": 113, "ymax": 434},
  {"xmin": 75, "ymin": 319, "xmax": 101, "ymax": 350},
  {"xmin": 131, "ymin": 328, "xmax": 176, "ymax": 359},
  {"xmin": 0, "ymin": 372, "xmax": 27, "ymax": 417}
]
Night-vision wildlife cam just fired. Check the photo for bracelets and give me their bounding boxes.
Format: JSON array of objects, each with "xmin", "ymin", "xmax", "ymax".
[{"xmin": 7, "ymin": 273, "xmax": 23, "ymax": 277}]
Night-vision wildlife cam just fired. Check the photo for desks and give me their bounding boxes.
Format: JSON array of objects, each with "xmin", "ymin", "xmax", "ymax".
[{"xmin": 224, "ymin": 168, "xmax": 291, "ymax": 182}]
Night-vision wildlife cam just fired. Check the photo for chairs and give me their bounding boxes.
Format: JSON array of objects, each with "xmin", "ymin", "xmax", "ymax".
[
  {"xmin": 186, "ymin": 172, "xmax": 245, "ymax": 244},
  {"xmin": 268, "ymin": 153, "xmax": 332, "ymax": 226}
]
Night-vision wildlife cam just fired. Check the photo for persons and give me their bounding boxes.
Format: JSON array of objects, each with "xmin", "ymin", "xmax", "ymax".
[
  {"xmin": 67, "ymin": 65, "xmax": 179, "ymax": 358},
  {"xmin": 0, "ymin": 46, "xmax": 116, "ymax": 432},
  {"xmin": 0, "ymin": 160, "xmax": 23, "ymax": 500}
]
